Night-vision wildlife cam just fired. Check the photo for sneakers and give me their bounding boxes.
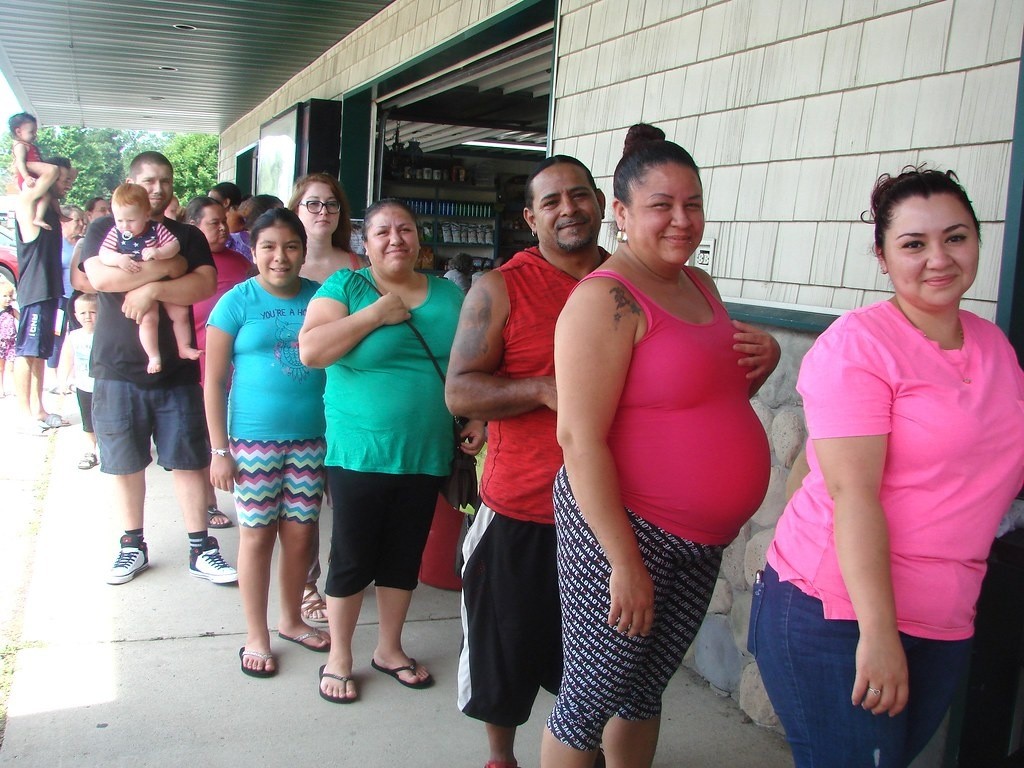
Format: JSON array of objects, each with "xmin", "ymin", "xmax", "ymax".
[
  {"xmin": 106, "ymin": 535, "xmax": 149, "ymax": 584},
  {"xmin": 189, "ymin": 536, "xmax": 238, "ymax": 584}
]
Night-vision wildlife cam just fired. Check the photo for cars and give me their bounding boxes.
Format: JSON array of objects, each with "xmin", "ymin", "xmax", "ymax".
[
  {"xmin": 0, "ymin": 225, "xmax": 16, "ymax": 246},
  {"xmin": 0, "ymin": 244, "xmax": 18, "ymax": 287}
]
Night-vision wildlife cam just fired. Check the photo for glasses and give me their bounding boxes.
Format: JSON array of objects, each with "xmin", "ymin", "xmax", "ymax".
[{"xmin": 298, "ymin": 200, "xmax": 341, "ymax": 214}]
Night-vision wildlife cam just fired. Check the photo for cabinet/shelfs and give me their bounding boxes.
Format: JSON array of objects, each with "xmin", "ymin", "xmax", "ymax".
[{"xmin": 381, "ymin": 175, "xmax": 539, "ymax": 275}]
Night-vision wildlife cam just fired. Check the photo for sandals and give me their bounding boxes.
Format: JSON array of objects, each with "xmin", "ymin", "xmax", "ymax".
[
  {"xmin": 207, "ymin": 506, "xmax": 232, "ymax": 528},
  {"xmin": 78, "ymin": 453, "xmax": 100, "ymax": 469},
  {"xmin": 301, "ymin": 583, "xmax": 328, "ymax": 622}
]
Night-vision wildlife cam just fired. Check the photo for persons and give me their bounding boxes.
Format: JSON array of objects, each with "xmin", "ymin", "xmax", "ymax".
[
  {"xmin": 0, "ymin": 283, "xmax": 20, "ymax": 401},
  {"xmin": 299, "ymin": 199, "xmax": 486, "ymax": 704},
  {"xmin": 77, "ymin": 151, "xmax": 241, "ymax": 585},
  {"xmin": 748, "ymin": 163, "xmax": 1024, "ymax": 768},
  {"xmin": 445, "ymin": 155, "xmax": 613, "ymax": 768},
  {"xmin": 14, "ymin": 157, "xmax": 78, "ymax": 436},
  {"xmin": 99, "ymin": 183, "xmax": 203, "ymax": 373},
  {"xmin": 204, "ymin": 207, "xmax": 332, "ymax": 679},
  {"xmin": 9, "ymin": 113, "xmax": 74, "ymax": 231},
  {"xmin": 540, "ymin": 123, "xmax": 781, "ymax": 768},
  {"xmin": 48, "ymin": 173, "xmax": 372, "ymax": 621},
  {"xmin": 443, "ymin": 253, "xmax": 507, "ymax": 296}
]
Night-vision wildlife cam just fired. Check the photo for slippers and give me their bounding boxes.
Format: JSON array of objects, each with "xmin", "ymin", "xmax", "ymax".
[
  {"xmin": 239, "ymin": 647, "xmax": 275, "ymax": 678},
  {"xmin": 17, "ymin": 414, "xmax": 71, "ymax": 435},
  {"xmin": 319, "ymin": 665, "xmax": 357, "ymax": 704},
  {"xmin": 279, "ymin": 627, "xmax": 330, "ymax": 653},
  {"xmin": 371, "ymin": 658, "xmax": 432, "ymax": 689}
]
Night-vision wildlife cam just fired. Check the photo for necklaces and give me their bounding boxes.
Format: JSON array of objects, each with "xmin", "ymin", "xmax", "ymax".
[{"xmin": 921, "ymin": 327, "xmax": 972, "ymax": 383}]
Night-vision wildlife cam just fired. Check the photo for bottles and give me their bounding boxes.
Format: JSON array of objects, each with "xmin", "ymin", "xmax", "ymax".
[
  {"xmin": 403, "ymin": 165, "xmax": 465, "ymax": 182},
  {"xmin": 400, "ymin": 196, "xmax": 492, "ymax": 218},
  {"xmin": 514, "ymin": 219, "xmax": 529, "ymax": 229}
]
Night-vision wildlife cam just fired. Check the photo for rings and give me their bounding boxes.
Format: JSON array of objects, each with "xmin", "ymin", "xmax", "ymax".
[{"xmin": 868, "ymin": 686, "xmax": 881, "ymax": 696}]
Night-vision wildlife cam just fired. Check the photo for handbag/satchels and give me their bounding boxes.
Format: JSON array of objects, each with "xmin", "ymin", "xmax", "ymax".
[{"xmin": 440, "ymin": 416, "xmax": 479, "ymax": 511}]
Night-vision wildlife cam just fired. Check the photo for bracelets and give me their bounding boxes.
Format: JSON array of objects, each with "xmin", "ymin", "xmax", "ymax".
[{"xmin": 210, "ymin": 447, "xmax": 230, "ymax": 457}]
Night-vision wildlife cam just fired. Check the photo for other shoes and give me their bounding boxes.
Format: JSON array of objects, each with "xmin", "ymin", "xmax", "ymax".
[
  {"xmin": 594, "ymin": 748, "xmax": 606, "ymax": 768},
  {"xmin": 0, "ymin": 392, "xmax": 7, "ymax": 398},
  {"xmin": 47, "ymin": 384, "xmax": 76, "ymax": 394},
  {"xmin": 484, "ymin": 760, "xmax": 517, "ymax": 768}
]
